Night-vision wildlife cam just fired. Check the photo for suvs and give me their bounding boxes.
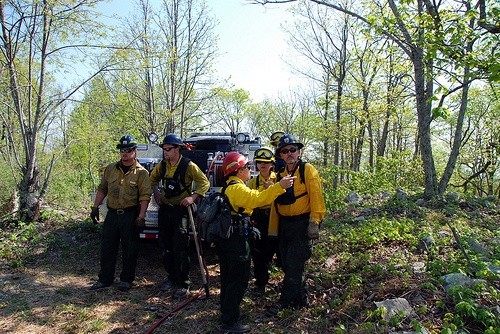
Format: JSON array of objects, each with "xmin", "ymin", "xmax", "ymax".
[{"xmin": 90, "ymin": 131, "xmax": 271, "ymax": 264}]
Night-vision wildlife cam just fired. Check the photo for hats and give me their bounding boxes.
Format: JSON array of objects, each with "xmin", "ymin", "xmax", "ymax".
[{"xmin": 275, "ymin": 133, "xmax": 304, "ymax": 157}]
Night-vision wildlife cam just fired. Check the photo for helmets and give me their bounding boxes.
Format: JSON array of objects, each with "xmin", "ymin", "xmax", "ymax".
[
  {"xmin": 158, "ymin": 133, "xmax": 184, "ymax": 147},
  {"xmin": 116, "ymin": 134, "xmax": 138, "ymax": 148},
  {"xmin": 268, "ymin": 130, "xmax": 286, "ymax": 146},
  {"xmin": 222, "ymin": 151, "xmax": 252, "ymax": 177},
  {"xmin": 253, "ymin": 145, "xmax": 277, "ymax": 163}
]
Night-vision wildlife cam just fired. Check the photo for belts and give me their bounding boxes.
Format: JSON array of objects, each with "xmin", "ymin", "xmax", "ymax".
[
  {"xmin": 107, "ymin": 205, "xmax": 140, "ymax": 215},
  {"xmin": 252, "ymin": 208, "xmax": 270, "ymax": 216}
]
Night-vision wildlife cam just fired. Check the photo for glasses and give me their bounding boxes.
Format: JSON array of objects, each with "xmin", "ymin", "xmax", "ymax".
[
  {"xmin": 162, "ymin": 146, "xmax": 175, "ymax": 151},
  {"xmin": 253, "ymin": 148, "xmax": 275, "ymax": 160},
  {"xmin": 270, "ymin": 131, "xmax": 285, "ymax": 141},
  {"xmin": 279, "ymin": 147, "xmax": 300, "ymax": 154},
  {"xmin": 120, "ymin": 146, "xmax": 136, "ymax": 153},
  {"xmin": 256, "ymin": 161, "xmax": 270, "ymax": 164},
  {"xmin": 247, "ymin": 163, "xmax": 250, "ymax": 170}
]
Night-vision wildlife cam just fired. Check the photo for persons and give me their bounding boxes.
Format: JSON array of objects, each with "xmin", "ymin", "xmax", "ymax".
[
  {"xmin": 89, "ymin": 135, "xmax": 152, "ymax": 291},
  {"xmin": 149, "ymin": 134, "xmax": 210, "ymax": 298},
  {"xmin": 217, "ymin": 131, "xmax": 325, "ymax": 333}
]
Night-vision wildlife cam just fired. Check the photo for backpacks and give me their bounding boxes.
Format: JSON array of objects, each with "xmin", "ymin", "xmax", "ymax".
[{"xmin": 194, "ymin": 179, "xmax": 246, "ymax": 256}]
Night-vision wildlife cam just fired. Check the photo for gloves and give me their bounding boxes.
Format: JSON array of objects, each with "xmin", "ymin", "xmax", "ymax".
[
  {"xmin": 135, "ymin": 216, "xmax": 146, "ymax": 234},
  {"xmin": 305, "ymin": 221, "xmax": 320, "ymax": 240},
  {"xmin": 90, "ymin": 207, "xmax": 99, "ymax": 224}
]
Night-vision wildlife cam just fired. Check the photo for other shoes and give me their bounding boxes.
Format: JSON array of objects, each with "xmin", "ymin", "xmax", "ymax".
[
  {"xmin": 89, "ymin": 281, "xmax": 110, "ymax": 290},
  {"xmin": 116, "ymin": 284, "xmax": 132, "ymax": 292},
  {"xmin": 220, "ymin": 320, "xmax": 251, "ymax": 334}
]
